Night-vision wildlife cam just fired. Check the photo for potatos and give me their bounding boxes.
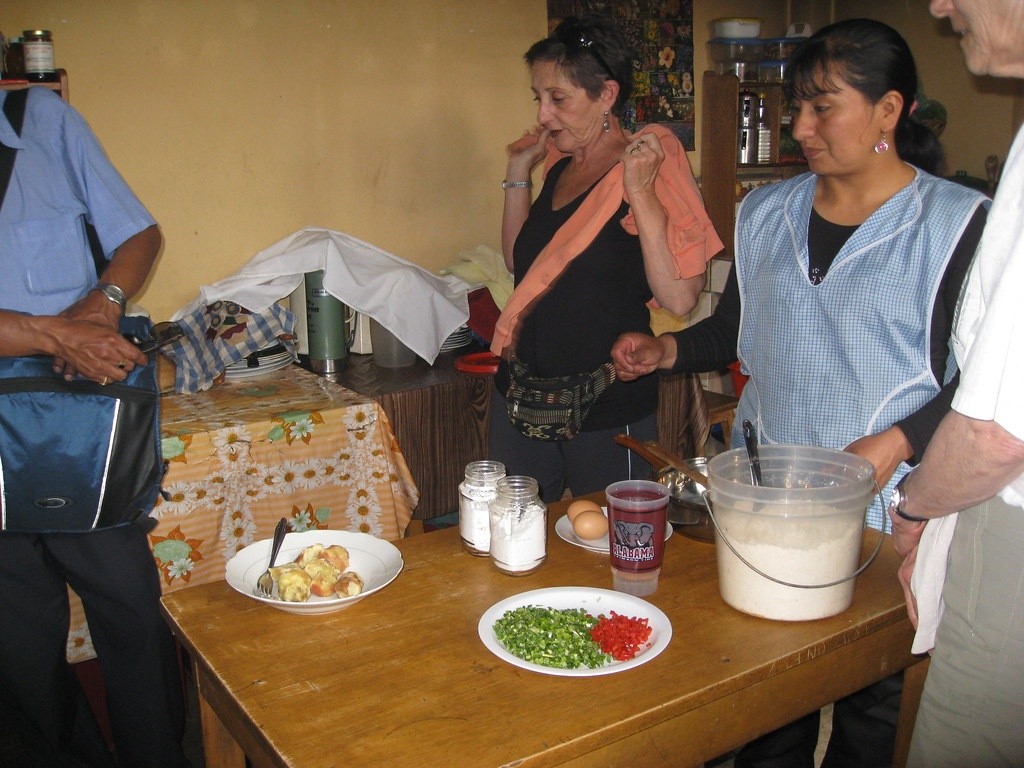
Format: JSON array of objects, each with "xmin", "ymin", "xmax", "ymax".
[{"xmin": 268, "ymin": 543, "xmax": 363, "ymax": 602}]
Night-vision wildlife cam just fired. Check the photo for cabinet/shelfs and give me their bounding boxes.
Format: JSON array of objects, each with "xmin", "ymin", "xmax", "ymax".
[{"xmin": 701, "ymin": 70, "xmax": 809, "ymax": 264}]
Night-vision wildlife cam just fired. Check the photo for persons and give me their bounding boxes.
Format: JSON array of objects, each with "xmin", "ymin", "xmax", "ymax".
[
  {"xmin": 886, "ymin": 0, "xmax": 1024, "ymax": 768},
  {"xmin": 487, "ymin": 11, "xmax": 707, "ymax": 506},
  {"xmin": 611, "ymin": 17, "xmax": 994, "ymax": 768},
  {"xmin": 0, "ymin": 84, "xmax": 193, "ymax": 768}
]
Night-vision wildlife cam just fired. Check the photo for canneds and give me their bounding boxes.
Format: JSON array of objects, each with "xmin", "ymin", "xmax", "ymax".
[{"xmin": 458, "ymin": 460, "xmax": 549, "ymax": 577}]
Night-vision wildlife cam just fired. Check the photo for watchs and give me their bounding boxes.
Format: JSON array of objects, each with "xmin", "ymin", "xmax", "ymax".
[
  {"xmin": 502, "ymin": 180, "xmax": 531, "ymax": 189},
  {"xmin": 890, "ymin": 471, "xmax": 928, "ymax": 521},
  {"xmin": 88, "ymin": 282, "xmax": 127, "ymax": 315}
]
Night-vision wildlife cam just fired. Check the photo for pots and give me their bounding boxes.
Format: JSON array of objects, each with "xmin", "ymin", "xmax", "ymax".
[{"xmin": 613, "ymin": 433, "xmax": 722, "ymax": 545}]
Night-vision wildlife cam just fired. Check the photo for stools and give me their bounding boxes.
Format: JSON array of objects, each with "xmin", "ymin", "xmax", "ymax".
[{"xmin": 704, "ymin": 390, "xmax": 740, "ymax": 453}]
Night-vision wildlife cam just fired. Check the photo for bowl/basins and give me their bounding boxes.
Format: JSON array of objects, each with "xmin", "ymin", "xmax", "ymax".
[{"xmin": 707, "ymin": 17, "xmax": 810, "ymax": 82}]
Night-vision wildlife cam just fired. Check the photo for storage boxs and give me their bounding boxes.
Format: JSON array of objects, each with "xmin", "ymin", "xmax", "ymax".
[{"xmin": 707, "ymin": 17, "xmax": 810, "ymax": 81}]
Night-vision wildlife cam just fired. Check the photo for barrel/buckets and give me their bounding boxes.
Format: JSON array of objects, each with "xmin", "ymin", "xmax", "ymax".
[{"xmin": 702, "ymin": 442, "xmax": 889, "ymax": 622}]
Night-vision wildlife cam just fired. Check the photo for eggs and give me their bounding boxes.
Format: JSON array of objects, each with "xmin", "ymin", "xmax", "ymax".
[
  {"xmin": 567, "ymin": 500, "xmax": 604, "ymax": 522},
  {"xmin": 574, "ymin": 509, "xmax": 609, "ymax": 540}
]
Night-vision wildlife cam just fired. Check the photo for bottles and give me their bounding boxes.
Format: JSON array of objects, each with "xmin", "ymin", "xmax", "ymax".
[
  {"xmin": 6, "ymin": 29, "xmax": 57, "ymax": 83},
  {"xmin": 754, "ymin": 92, "xmax": 771, "ymax": 166},
  {"xmin": 457, "ymin": 460, "xmax": 506, "ymax": 557},
  {"xmin": 302, "ymin": 271, "xmax": 359, "ymax": 373},
  {"xmin": 489, "ymin": 474, "xmax": 548, "ymax": 577}
]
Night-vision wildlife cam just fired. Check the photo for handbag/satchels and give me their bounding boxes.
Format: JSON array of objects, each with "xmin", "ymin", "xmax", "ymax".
[
  {"xmin": 507, "ymin": 363, "xmax": 606, "ymax": 443},
  {"xmin": 0, "ymin": 315, "xmax": 164, "ymax": 537}
]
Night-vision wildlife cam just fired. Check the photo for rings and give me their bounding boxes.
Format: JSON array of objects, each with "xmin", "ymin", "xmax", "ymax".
[
  {"xmin": 117, "ymin": 357, "xmax": 126, "ymax": 369},
  {"xmin": 631, "ymin": 148, "xmax": 638, "ymax": 154},
  {"xmin": 99, "ymin": 377, "xmax": 107, "ymax": 386},
  {"xmin": 637, "ymin": 140, "xmax": 646, "ymax": 150}
]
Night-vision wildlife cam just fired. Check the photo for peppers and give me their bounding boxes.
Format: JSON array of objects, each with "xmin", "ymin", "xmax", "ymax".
[{"xmin": 586, "ymin": 609, "xmax": 653, "ymax": 660}]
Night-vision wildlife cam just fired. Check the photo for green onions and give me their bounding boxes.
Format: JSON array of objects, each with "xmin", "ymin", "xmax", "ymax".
[{"xmin": 492, "ymin": 604, "xmax": 612, "ymax": 668}]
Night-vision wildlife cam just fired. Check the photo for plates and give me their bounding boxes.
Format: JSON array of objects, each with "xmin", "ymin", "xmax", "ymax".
[
  {"xmin": 223, "ymin": 529, "xmax": 404, "ymax": 615},
  {"xmin": 221, "ymin": 333, "xmax": 301, "ymax": 380},
  {"xmin": 555, "ymin": 506, "xmax": 674, "ymax": 554},
  {"xmin": 434, "ymin": 324, "xmax": 475, "ymax": 354},
  {"xmin": 477, "ymin": 584, "xmax": 673, "ymax": 677}
]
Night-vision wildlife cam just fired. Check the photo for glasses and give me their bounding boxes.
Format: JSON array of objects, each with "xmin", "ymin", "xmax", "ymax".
[{"xmin": 551, "ymin": 24, "xmax": 622, "ymax": 96}]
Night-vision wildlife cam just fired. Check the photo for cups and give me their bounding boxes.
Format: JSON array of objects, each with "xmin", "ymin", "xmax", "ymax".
[{"xmin": 605, "ymin": 480, "xmax": 670, "ymax": 597}]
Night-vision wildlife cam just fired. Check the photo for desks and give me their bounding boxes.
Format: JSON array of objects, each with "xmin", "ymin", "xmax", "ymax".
[
  {"xmin": 298, "ymin": 358, "xmax": 712, "ymax": 526},
  {"xmin": 60, "ymin": 365, "xmax": 421, "ymax": 751},
  {"xmin": 158, "ymin": 492, "xmax": 931, "ymax": 768}
]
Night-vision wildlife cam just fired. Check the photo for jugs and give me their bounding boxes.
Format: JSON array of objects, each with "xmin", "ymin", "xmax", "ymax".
[{"xmin": 368, "ymin": 315, "xmax": 417, "ymax": 368}]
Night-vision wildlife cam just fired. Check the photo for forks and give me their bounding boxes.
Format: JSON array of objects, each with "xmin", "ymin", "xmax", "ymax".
[{"xmin": 256, "ymin": 517, "xmax": 287, "ymax": 595}]
